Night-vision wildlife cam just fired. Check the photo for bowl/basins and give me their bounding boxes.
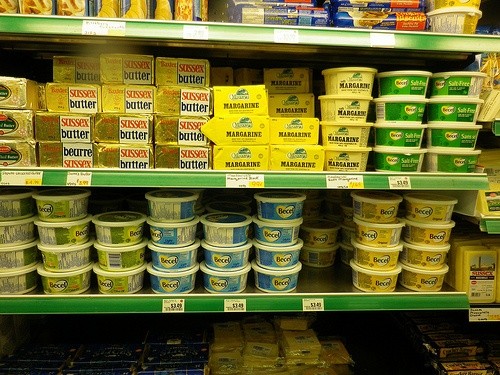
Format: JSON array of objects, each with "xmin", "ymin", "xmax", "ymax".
[
  {"xmin": 318, "ymin": 64, "xmax": 487, "ymax": 174},
  {"xmin": 425, "ymin": 0, "xmax": 482, "ymax": 34},
  {"xmin": 0, "ymin": 185, "xmax": 458, "ymax": 292}
]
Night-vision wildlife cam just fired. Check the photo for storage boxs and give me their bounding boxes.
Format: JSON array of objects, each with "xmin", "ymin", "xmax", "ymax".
[
  {"xmin": 418, "ymin": 323, "xmax": 500, "ymax": 375},
  {"xmin": 0, "ymin": 54, "xmax": 325, "ymax": 171}
]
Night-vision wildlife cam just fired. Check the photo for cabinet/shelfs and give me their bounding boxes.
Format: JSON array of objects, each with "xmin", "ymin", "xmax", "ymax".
[{"xmin": 0, "ymin": 14, "xmax": 500, "ymax": 313}]
